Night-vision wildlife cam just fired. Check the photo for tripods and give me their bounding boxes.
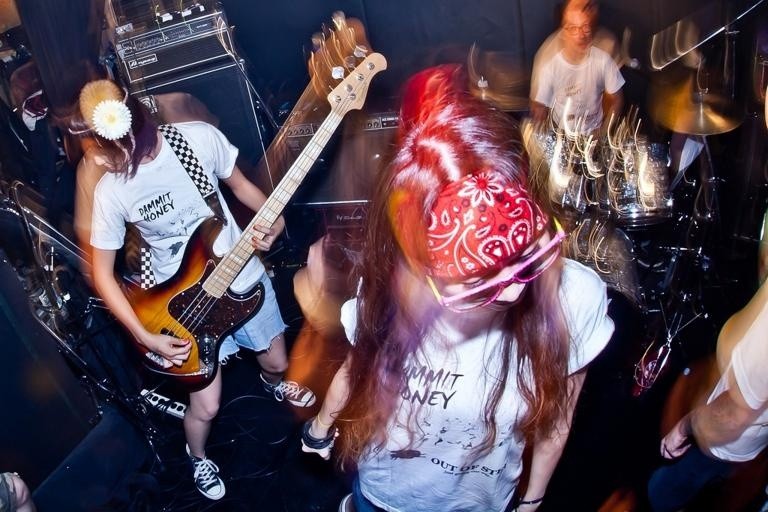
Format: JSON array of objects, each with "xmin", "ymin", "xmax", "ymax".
[{"xmin": 637, "ymin": 133, "xmax": 717, "ymax": 346}]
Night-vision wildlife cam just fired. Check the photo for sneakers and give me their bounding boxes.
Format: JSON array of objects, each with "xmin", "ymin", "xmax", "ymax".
[
  {"xmin": 183, "ymin": 445, "xmax": 227, "ymax": 502},
  {"xmin": 258, "ymin": 369, "xmax": 317, "ymax": 410}
]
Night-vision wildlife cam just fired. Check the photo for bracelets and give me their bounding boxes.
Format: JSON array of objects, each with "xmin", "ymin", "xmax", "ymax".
[
  {"xmin": 299, "ymin": 416, "xmax": 337, "ymax": 449},
  {"xmin": 516, "ymin": 494, "xmax": 546, "ymax": 507}
]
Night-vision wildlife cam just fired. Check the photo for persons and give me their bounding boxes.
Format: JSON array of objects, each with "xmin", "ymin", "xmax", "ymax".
[
  {"xmin": 602, "ymin": 275, "xmax": 767, "ymax": 512},
  {"xmin": 0, "ymin": 471, "xmax": 37, "ymax": 511},
  {"xmin": 297, "ymin": 60, "xmax": 616, "ymax": 512},
  {"xmin": 523, "ymin": 0, "xmax": 630, "ymax": 152},
  {"xmin": 60, "ymin": 75, "xmax": 318, "ymax": 500}
]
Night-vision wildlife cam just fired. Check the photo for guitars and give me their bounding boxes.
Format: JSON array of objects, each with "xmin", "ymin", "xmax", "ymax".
[{"xmin": 130, "ymin": 45, "xmax": 387, "ymax": 392}]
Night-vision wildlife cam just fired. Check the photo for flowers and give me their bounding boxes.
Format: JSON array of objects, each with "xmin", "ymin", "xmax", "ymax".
[{"xmin": 93, "ymin": 100, "xmax": 132, "ymax": 140}]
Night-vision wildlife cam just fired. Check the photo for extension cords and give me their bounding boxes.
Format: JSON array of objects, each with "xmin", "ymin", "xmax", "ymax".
[{"xmin": 141, "ymin": 388, "xmax": 187, "ymax": 420}]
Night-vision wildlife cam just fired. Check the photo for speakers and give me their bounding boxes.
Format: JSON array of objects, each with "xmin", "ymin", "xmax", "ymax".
[
  {"xmin": 280, "ymin": 111, "xmax": 404, "ymax": 205},
  {"xmin": 125, "ymin": 59, "xmax": 291, "ymax": 262}
]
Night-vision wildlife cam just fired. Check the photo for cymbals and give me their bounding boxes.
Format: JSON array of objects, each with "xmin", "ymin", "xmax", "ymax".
[{"xmin": 652, "ymin": 75, "xmax": 743, "ymax": 135}]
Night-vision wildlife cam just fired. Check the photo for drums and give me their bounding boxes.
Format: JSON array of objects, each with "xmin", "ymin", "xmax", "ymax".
[
  {"xmin": 589, "ymin": 136, "xmax": 673, "ymax": 225},
  {"xmin": 557, "ymin": 212, "xmax": 643, "ymax": 307}
]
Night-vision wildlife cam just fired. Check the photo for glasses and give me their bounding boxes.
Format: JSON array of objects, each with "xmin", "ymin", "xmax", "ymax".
[{"xmin": 412, "ymin": 205, "xmax": 566, "ymax": 317}]
